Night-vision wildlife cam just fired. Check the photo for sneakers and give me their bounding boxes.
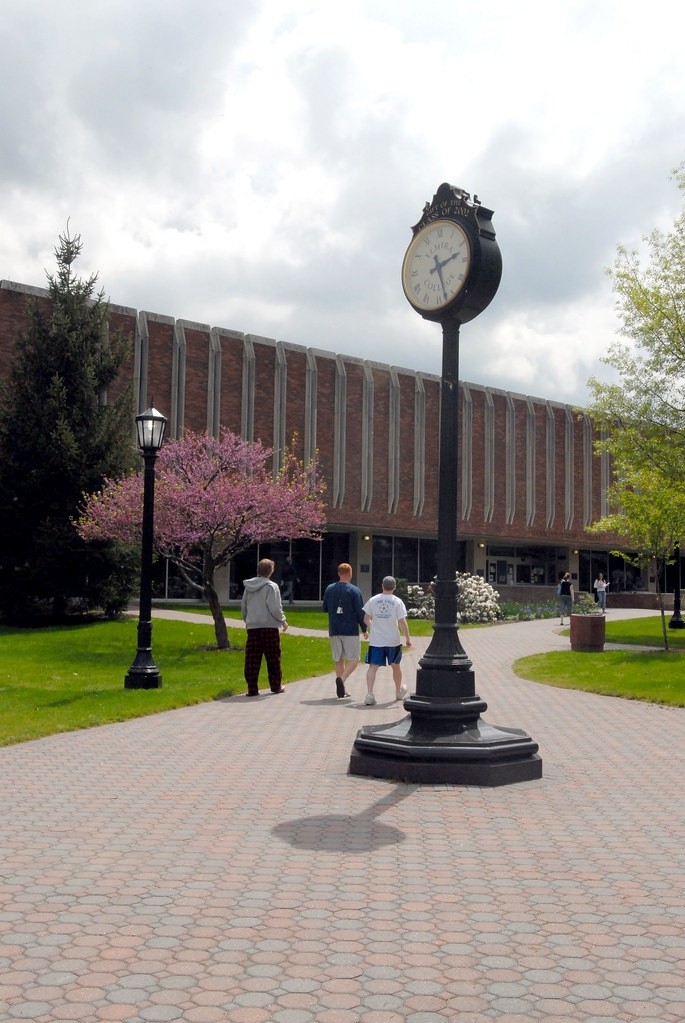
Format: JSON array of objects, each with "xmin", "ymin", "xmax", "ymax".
[
  {"xmin": 364, "ymin": 695, "xmax": 377, "ymax": 705},
  {"xmin": 395, "ymin": 684, "xmax": 408, "ymax": 700}
]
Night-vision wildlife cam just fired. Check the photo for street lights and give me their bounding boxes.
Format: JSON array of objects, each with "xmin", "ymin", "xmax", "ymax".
[{"xmin": 132, "ymin": 400, "xmax": 166, "ymax": 670}]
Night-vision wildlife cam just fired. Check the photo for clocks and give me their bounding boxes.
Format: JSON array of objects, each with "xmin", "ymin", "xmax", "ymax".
[{"xmin": 401, "ymin": 220, "xmax": 470, "ymax": 311}]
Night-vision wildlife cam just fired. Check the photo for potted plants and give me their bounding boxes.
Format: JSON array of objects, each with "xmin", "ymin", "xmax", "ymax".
[{"xmin": 570, "ymin": 594, "xmax": 606, "ymax": 653}]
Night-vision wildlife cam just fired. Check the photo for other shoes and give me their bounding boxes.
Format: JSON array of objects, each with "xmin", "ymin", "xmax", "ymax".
[
  {"xmin": 270, "ymin": 685, "xmax": 285, "ymax": 694},
  {"xmin": 335, "ymin": 676, "xmax": 345, "ymax": 698}
]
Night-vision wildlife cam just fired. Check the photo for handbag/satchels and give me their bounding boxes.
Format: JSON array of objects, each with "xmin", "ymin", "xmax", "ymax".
[
  {"xmin": 594, "ymin": 580, "xmax": 599, "ymax": 588},
  {"xmin": 556, "ymin": 584, "xmax": 561, "ymax": 596}
]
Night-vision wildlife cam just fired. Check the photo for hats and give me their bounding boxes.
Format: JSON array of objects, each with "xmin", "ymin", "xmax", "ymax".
[{"xmin": 383, "ymin": 576, "xmax": 396, "ymax": 587}]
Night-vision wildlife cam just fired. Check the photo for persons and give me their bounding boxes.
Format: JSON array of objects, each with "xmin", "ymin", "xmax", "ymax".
[
  {"xmin": 361, "ymin": 576, "xmax": 412, "ymax": 705},
  {"xmin": 281, "ymin": 557, "xmax": 300, "ymax": 605},
  {"xmin": 241, "ymin": 559, "xmax": 289, "ymax": 697},
  {"xmin": 559, "ymin": 573, "xmax": 574, "ymax": 626},
  {"xmin": 593, "ymin": 573, "xmax": 610, "ymax": 613},
  {"xmin": 321, "ymin": 563, "xmax": 369, "ymax": 699}
]
